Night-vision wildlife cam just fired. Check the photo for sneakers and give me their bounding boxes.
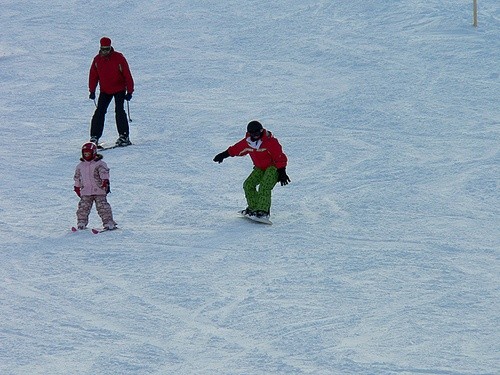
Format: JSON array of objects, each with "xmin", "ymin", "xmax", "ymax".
[
  {"xmin": 77, "ymin": 223, "xmax": 86, "ymax": 230},
  {"xmin": 104, "ymin": 222, "xmax": 117, "ymax": 229},
  {"xmin": 241, "ymin": 209, "xmax": 270, "ymax": 218},
  {"xmin": 115, "ymin": 135, "xmax": 131, "ymax": 146},
  {"xmin": 89, "ymin": 137, "xmax": 104, "ymax": 151}
]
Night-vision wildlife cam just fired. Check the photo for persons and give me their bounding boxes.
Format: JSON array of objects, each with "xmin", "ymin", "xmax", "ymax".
[
  {"xmin": 88, "ymin": 37, "xmax": 134, "ymax": 149},
  {"xmin": 74, "ymin": 142, "xmax": 115, "ymax": 230},
  {"xmin": 213, "ymin": 120, "xmax": 291, "ymax": 218}
]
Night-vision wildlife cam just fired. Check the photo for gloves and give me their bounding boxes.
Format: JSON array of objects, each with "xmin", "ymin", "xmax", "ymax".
[
  {"xmin": 213, "ymin": 150, "xmax": 230, "ymax": 164},
  {"xmin": 101, "ymin": 180, "xmax": 110, "ymax": 194},
  {"xmin": 73, "ymin": 186, "xmax": 81, "ymax": 197},
  {"xmin": 277, "ymin": 167, "xmax": 292, "ymax": 186},
  {"xmin": 89, "ymin": 92, "xmax": 95, "ymax": 99},
  {"xmin": 124, "ymin": 93, "xmax": 132, "ymax": 101}
]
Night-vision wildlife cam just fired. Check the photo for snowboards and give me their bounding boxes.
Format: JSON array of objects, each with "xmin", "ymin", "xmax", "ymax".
[{"xmin": 239, "ymin": 211, "xmax": 273, "ymax": 224}]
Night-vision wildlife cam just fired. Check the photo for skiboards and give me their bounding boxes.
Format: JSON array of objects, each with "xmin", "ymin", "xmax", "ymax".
[
  {"xmin": 97, "ymin": 142, "xmax": 135, "ymax": 150},
  {"xmin": 71, "ymin": 225, "xmax": 120, "ymax": 234}
]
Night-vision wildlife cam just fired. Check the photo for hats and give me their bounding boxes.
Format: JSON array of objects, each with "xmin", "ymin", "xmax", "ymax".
[
  {"xmin": 247, "ymin": 120, "xmax": 263, "ymax": 135},
  {"xmin": 100, "ymin": 37, "xmax": 112, "ymax": 46}
]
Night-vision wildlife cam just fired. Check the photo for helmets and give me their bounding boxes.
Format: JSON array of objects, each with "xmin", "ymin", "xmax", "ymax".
[{"xmin": 82, "ymin": 142, "xmax": 97, "ymax": 156}]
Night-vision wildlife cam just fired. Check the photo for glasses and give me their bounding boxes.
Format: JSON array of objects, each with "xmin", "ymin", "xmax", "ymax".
[{"xmin": 101, "ymin": 48, "xmax": 110, "ymax": 51}]
